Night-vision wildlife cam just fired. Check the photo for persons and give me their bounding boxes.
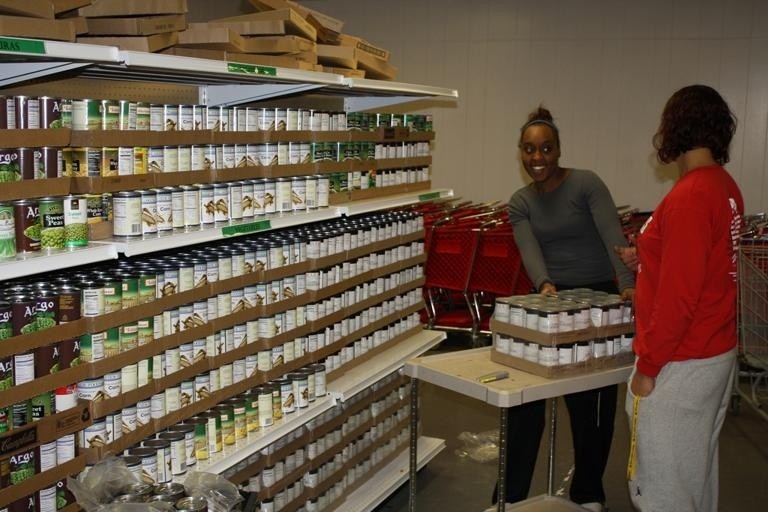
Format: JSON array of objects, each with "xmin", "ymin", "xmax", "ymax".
[
  {"xmin": 491, "ymin": 104, "xmax": 635, "ymax": 512},
  {"xmin": 614, "ymin": 84, "xmax": 745, "ymax": 512}
]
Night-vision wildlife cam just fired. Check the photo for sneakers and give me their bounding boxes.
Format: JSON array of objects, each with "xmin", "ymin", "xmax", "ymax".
[
  {"xmin": 483, "ymin": 502, "xmax": 510, "ymax": 512},
  {"xmin": 582, "ymin": 502, "xmax": 602, "ymax": 512}
]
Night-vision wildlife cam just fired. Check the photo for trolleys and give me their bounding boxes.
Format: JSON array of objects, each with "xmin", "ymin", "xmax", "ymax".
[
  {"xmin": 727, "ymin": 218, "xmax": 768, "ymax": 421},
  {"xmin": 418, "ymin": 195, "xmax": 652, "ymax": 350}
]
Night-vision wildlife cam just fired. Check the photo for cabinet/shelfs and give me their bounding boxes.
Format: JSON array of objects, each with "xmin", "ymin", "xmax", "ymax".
[
  {"xmin": 220, "ymin": 77, "xmax": 459, "ymax": 512},
  {"xmin": 0, "ymin": 49, "xmax": 349, "ymax": 508},
  {"xmin": 0, "ymin": 38, "xmax": 122, "ymax": 512}
]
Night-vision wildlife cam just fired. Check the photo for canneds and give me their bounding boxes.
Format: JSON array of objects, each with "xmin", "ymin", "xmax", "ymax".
[
  {"xmin": 493, "ymin": 286, "xmax": 635, "ymax": 334},
  {"xmin": 0, "ymin": 139, "xmax": 432, "ymax": 185},
  {"xmin": 0, "ymin": 94, "xmax": 435, "ymax": 134},
  {"xmin": 490, "ymin": 331, "xmax": 634, "ymax": 369},
  {"xmin": 0, "ymin": 174, "xmax": 428, "ymax": 512}
]
